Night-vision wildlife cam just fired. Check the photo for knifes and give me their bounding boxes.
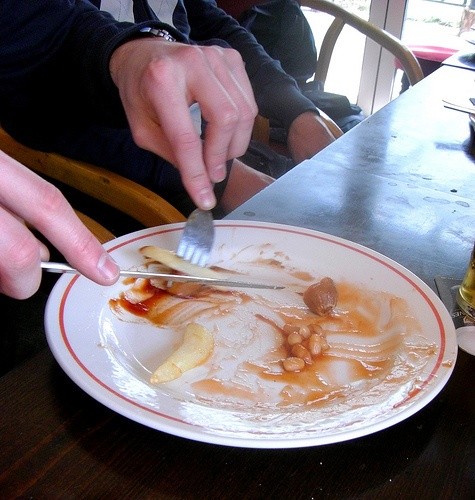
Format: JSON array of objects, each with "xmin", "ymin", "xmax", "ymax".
[{"xmin": 40, "ymin": 261, "xmax": 286, "ymax": 291}]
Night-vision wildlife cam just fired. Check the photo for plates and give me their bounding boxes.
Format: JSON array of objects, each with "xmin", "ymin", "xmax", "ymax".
[{"xmin": 44, "ymin": 219, "xmax": 457, "ymax": 449}]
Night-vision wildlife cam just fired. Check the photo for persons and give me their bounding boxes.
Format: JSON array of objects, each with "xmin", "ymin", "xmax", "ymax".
[
  {"xmin": 0, "ymin": 0, "xmax": 259, "ymax": 300},
  {"xmin": 91, "ymin": 0, "xmax": 336, "ymax": 218}
]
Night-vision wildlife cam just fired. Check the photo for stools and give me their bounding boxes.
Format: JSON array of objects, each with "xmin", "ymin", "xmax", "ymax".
[{"xmin": 394, "ymin": 46, "xmax": 459, "ymax": 97}]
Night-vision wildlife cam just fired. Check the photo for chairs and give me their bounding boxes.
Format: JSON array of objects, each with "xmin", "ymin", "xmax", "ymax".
[
  {"xmin": 0, "ymin": 128, "xmax": 187, "ymax": 227},
  {"xmin": 296, "ymin": 0, "xmax": 424, "ymax": 136}
]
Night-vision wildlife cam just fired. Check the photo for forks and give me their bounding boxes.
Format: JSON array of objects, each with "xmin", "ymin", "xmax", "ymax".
[{"xmin": 174, "ymin": 180, "xmax": 216, "ymax": 270}]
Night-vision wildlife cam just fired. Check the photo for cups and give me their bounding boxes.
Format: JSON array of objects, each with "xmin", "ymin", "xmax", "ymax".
[{"xmin": 457, "ymin": 243, "xmax": 475, "ymax": 323}]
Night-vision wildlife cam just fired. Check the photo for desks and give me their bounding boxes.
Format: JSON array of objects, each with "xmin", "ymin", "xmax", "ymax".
[{"xmin": 0, "ymin": 55, "xmax": 475, "ymax": 500}]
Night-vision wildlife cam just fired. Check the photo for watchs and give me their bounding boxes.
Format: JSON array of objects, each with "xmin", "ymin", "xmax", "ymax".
[{"xmin": 140, "ymin": 27, "xmax": 176, "ymax": 42}]
